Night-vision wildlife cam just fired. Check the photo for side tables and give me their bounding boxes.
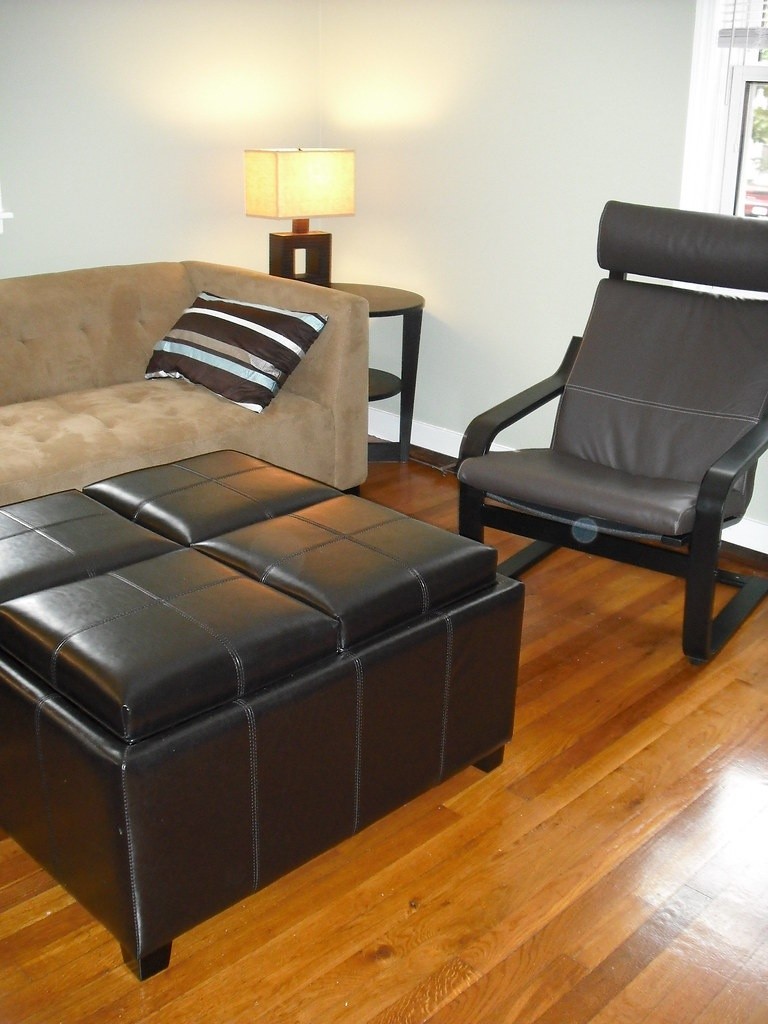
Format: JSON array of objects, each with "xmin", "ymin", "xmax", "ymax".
[{"xmin": 330, "ymin": 283, "xmax": 425, "ymax": 464}]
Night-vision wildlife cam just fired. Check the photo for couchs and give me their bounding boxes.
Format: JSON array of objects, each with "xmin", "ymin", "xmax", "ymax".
[{"xmin": 0, "ymin": 261, "xmax": 369, "ymax": 507}]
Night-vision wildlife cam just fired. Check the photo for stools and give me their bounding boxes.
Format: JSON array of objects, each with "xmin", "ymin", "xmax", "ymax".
[{"xmin": 0, "ymin": 448, "xmax": 526, "ymax": 982}]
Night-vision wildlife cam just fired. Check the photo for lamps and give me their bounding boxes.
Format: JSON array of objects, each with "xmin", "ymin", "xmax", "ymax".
[{"xmin": 244, "ymin": 147, "xmax": 356, "ymax": 288}]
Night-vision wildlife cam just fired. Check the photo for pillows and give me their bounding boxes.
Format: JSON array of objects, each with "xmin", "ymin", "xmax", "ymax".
[{"xmin": 144, "ymin": 291, "xmax": 329, "ymax": 413}]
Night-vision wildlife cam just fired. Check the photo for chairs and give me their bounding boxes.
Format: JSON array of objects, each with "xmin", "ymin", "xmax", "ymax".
[{"xmin": 457, "ymin": 202, "xmax": 768, "ymax": 665}]
